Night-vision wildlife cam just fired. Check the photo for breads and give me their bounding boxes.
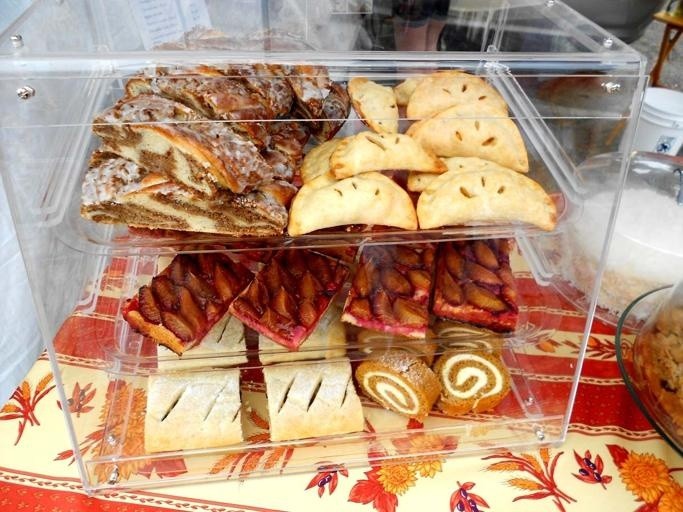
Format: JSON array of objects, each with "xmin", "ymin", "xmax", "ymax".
[{"xmin": 79, "ymin": 24, "xmax": 557, "ymax": 456}]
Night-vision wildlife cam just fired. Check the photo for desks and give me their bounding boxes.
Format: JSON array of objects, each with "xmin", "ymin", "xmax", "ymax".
[{"xmin": 1, "ymin": 208, "xmax": 683, "ymax": 510}]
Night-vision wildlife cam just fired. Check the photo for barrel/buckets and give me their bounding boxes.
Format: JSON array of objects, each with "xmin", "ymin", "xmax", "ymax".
[{"xmin": 619, "ymin": 86, "xmax": 683, "ymax": 158}]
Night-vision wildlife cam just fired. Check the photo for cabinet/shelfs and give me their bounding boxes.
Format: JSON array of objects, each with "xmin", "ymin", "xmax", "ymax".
[{"xmin": 0, "ymin": 0, "xmax": 653, "ymax": 496}]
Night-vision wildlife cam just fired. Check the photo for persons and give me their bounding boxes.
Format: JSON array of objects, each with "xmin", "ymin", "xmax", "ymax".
[{"xmin": 391, "ymin": 0, "xmax": 451, "ymax": 86}]
